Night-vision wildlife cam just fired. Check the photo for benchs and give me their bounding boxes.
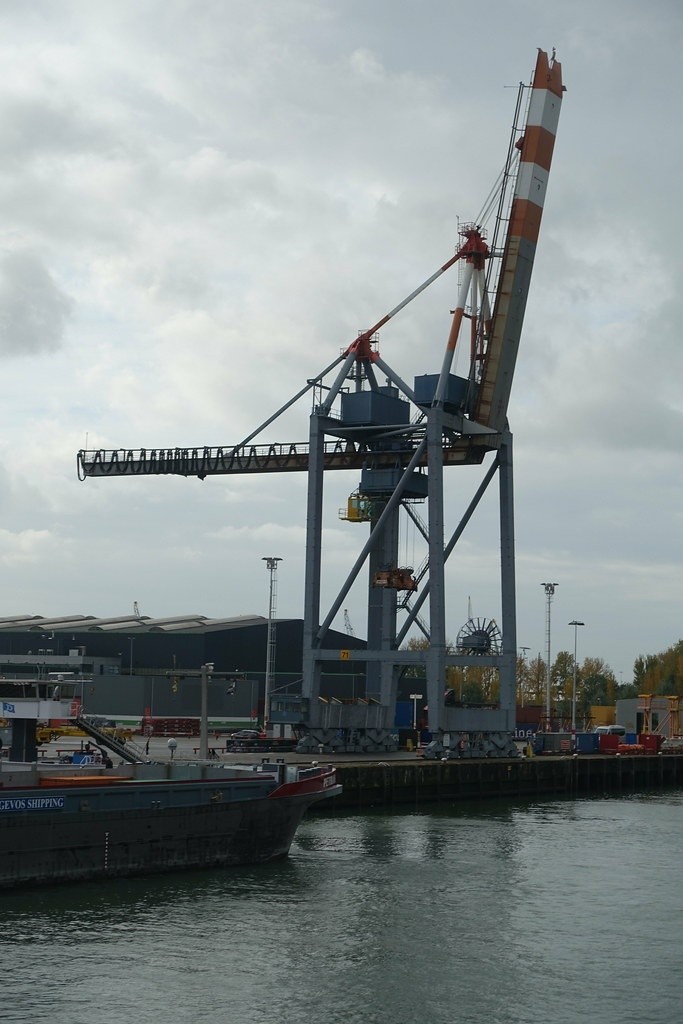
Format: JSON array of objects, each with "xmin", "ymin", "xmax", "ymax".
[
  {"xmin": 232, "ymin": 747, "xmax": 264, "ymax": 753},
  {"xmin": 192, "ymin": 748, "xmax": 227, "ymax": 754},
  {"xmin": 56, "ymin": 749, "xmax": 97, "ymax": 756},
  {"xmin": 1, "ymin": 748, "xmax": 48, "ymax": 757}
]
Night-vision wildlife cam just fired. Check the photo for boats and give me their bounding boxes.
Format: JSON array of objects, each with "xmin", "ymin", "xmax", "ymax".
[{"xmin": 1, "ymin": 761, "xmax": 345, "ymax": 887}]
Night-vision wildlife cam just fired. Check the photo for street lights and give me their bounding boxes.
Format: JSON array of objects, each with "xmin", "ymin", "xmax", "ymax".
[
  {"xmin": 261, "ymin": 555, "xmax": 283, "ymax": 735},
  {"xmin": 569, "ymin": 620, "xmax": 585, "ymax": 733},
  {"xmin": 541, "ymin": 581, "xmax": 559, "ymax": 733}
]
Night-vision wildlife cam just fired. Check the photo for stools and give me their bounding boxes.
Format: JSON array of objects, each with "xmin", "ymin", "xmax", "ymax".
[
  {"xmin": 276, "ymin": 759, "xmax": 284, "ymax": 763},
  {"xmin": 262, "ymin": 758, "xmax": 270, "ymax": 763}
]
